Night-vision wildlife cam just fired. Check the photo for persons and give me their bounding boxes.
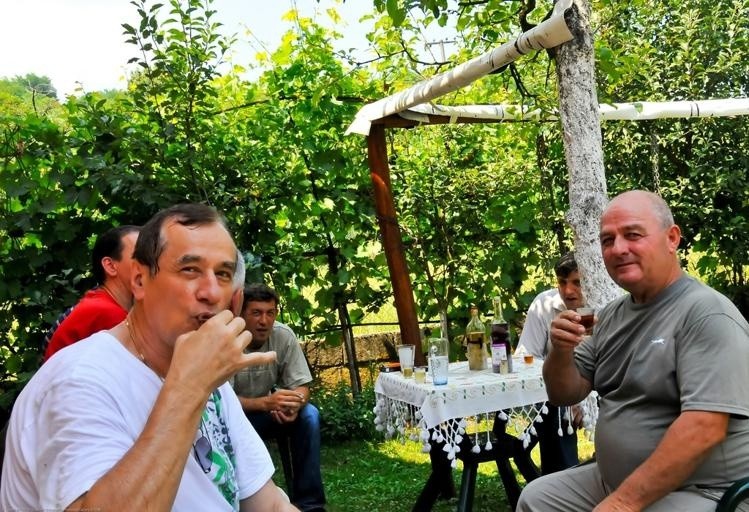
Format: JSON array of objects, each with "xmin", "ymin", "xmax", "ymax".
[
  {"xmin": 514, "ymin": 252, "xmax": 588, "ymax": 431},
  {"xmin": 515, "ymin": 190, "xmax": 749, "ymax": 512},
  {"xmin": 43, "ymin": 305, "xmax": 76, "ymax": 348},
  {"xmin": 43, "ymin": 226, "xmax": 141, "ymax": 362},
  {"xmin": 1, "ymin": 202, "xmax": 299, "ymax": 512},
  {"xmin": 226, "ymin": 283, "xmax": 326, "ymax": 511}
]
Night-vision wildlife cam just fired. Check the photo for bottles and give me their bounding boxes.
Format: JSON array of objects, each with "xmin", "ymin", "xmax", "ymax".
[
  {"xmin": 488, "ymin": 296, "xmax": 514, "ymax": 373},
  {"xmin": 465, "ymin": 306, "xmax": 488, "ymax": 372}
]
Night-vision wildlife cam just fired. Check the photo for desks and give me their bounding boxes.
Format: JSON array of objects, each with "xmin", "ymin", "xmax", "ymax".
[{"xmin": 375, "ymin": 355, "xmax": 562, "ymax": 511}]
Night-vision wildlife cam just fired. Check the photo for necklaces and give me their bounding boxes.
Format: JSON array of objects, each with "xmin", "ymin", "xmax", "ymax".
[{"xmin": 126, "ymin": 317, "xmax": 152, "ymax": 367}]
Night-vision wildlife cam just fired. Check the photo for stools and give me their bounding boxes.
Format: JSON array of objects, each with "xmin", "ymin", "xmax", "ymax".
[{"xmin": 274, "ymin": 432, "xmax": 298, "ymax": 497}]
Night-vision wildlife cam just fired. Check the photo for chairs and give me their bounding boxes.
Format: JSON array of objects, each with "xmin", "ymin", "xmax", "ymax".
[{"xmin": 715, "ymin": 478, "xmax": 749, "ymax": 512}]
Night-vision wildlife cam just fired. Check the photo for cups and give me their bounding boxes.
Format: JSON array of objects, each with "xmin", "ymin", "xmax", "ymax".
[
  {"xmin": 576, "ymin": 306, "xmax": 594, "ymax": 335},
  {"xmin": 397, "ymin": 338, "xmax": 534, "ymax": 386}
]
